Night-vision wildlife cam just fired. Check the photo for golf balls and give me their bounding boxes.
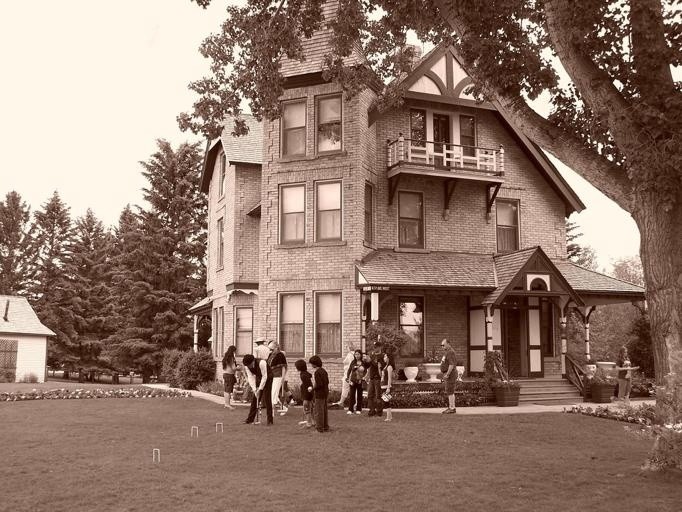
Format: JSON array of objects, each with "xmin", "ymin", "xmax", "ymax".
[{"xmin": 280, "ymin": 412, "xmax": 284, "ymax": 416}]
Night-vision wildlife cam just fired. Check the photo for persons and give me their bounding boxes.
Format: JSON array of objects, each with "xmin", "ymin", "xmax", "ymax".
[
  {"xmin": 438, "ymin": 338, "xmax": 460, "ymax": 415},
  {"xmin": 220, "ymin": 336, "xmax": 399, "ymax": 434},
  {"xmin": 614, "ymin": 344, "xmax": 640, "ymax": 405}
]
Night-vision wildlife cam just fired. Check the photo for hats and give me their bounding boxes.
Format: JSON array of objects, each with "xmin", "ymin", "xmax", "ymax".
[
  {"xmin": 374, "ymin": 341, "xmax": 382, "ymax": 347},
  {"xmin": 242, "ymin": 355, "xmax": 254, "ymax": 365},
  {"xmin": 253, "ymin": 338, "xmax": 265, "ymax": 342}
]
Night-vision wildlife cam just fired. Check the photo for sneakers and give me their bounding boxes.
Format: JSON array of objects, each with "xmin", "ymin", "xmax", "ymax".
[
  {"xmin": 280, "ymin": 406, "xmax": 288, "ymax": 416},
  {"xmin": 347, "ymin": 411, "xmax": 352, "ymax": 415},
  {"xmin": 355, "ymin": 411, "xmax": 361, "ymax": 415},
  {"xmin": 442, "ymin": 408, "xmax": 456, "ymax": 414}
]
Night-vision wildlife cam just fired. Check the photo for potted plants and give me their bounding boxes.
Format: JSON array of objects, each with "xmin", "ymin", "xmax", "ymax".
[
  {"xmin": 582, "ymin": 355, "xmax": 619, "ymax": 403},
  {"xmin": 404, "ymin": 344, "xmax": 521, "ymax": 406}
]
